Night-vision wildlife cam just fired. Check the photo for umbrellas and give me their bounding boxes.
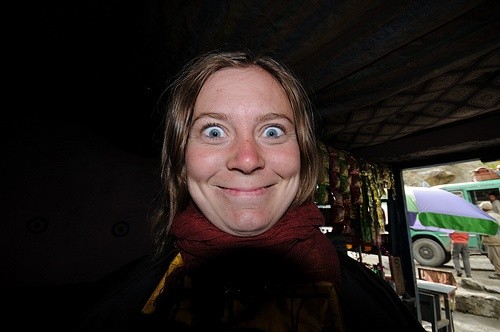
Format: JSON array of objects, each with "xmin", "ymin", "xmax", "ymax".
[{"xmin": 401, "ymin": 182, "xmax": 500, "ymax": 237}]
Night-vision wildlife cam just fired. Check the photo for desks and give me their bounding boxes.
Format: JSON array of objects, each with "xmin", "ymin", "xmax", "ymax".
[{"xmin": 416, "ymin": 279, "xmax": 458, "ymax": 332}]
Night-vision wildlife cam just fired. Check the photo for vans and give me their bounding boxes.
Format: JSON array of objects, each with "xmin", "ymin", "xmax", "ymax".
[{"xmin": 410, "ymin": 179, "xmax": 500, "ymax": 266}]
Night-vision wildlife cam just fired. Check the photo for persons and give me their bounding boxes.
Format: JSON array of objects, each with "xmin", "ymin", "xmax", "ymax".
[
  {"xmin": 449, "ymin": 231, "xmax": 472, "ymax": 279},
  {"xmin": 489, "ymin": 195, "xmax": 499, "ymax": 214},
  {"xmin": 474, "ymin": 200, "xmax": 500, "ymax": 279},
  {"xmin": 70, "ymin": 49, "xmax": 430, "ymax": 332}
]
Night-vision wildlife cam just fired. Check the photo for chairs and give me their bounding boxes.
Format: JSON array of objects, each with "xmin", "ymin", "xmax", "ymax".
[{"xmin": 418, "ymin": 292, "xmax": 450, "ymax": 332}]
